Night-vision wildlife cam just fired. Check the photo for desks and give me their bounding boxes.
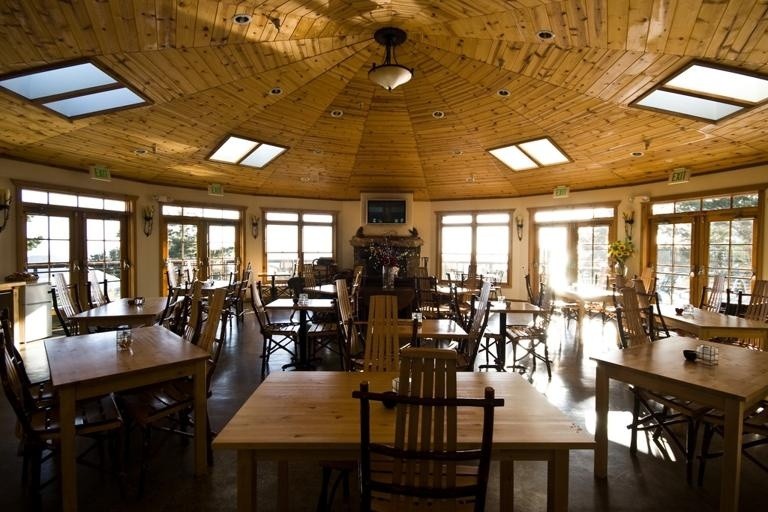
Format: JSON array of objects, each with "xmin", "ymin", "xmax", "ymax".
[
  {"xmin": 302, "ymin": 283, "xmax": 350, "ymax": 312},
  {"xmin": 71, "ymin": 296, "xmax": 189, "ymax": 334},
  {"xmin": 210, "ymin": 371, "xmax": 596, "ymax": 512},
  {"xmin": 541, "ymin": 282, "xmax": 621, "ymax": 325},
  {"xmin": 425, "ymin": 285, "xmax": 495, "ymax": 309},
  {"xmin": 636, "ymin": 303, "xmax": 767, "ymax": 351},
  {"xmin": 374, "ymin": 319, "xmax": 470, "ymax": 371},
  {"xmin": 1, "ymin": 280, "xmax": 25, "ymax": 351},
  {"xmin": 264, "ymin": 298, "xmax": 340, "ymax": 370},
  {"xmin": 44, "ymin": 324, "xmax": 212, "ymax": 512},
  {"xmin": 465, "ymin": 300, "xmax": 546, "ymax": 366},
  {"xmin": 589, "ymin": 335, "xmax": 767, "ymax": 512},
  {"xmin": 172, "ymin": 278, "xmax": 240, "ymax": 330},
  {"xmin": 256, "ymin": 271, "xmax": 291, "ymax": 302},
  {"xmin": 356, "ymin": 286, "xmax": 415, "ymax": 319}
]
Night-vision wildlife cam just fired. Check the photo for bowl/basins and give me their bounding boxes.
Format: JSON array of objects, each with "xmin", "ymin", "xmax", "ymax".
[
  {"xmin": 674, "ymin": 308, "xmax": 684, "ymax": 315},
  {"xmin": 682, "ymin": 349, "xmax": 696, "ymax": 362}
]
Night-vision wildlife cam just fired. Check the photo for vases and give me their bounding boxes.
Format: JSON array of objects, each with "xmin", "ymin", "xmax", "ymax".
[
  {"xmin": 382, "ymin": 266, "xmax": 395, "ymax": 289},
  {"xmin": 613, "ymin": 258, "xmax": 627, "ymax": 278}
]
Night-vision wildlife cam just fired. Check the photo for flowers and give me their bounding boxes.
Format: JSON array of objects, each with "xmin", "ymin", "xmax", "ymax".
[
  {"xmin": 361, "ymin": 232, "xmax": 420, "ymax": 273},
  {"xmin": 607, "ymin": 239, "xmax": 635, "ymax": 262}
]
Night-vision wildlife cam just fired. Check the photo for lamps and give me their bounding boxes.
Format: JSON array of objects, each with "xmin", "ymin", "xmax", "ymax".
[
  {"xmin": 143, "ymin": 203, "xmax": 155, "ymax": 237},
  {"xmin": 622, "ymin": 210, "xmax": 634, "ymax": 240},
  {"xmin": 0, "ymin": 189, "xmax": 12, "ymax": 233},
  {"xmin": 516, "ymin": 215, "xmax": 525, "ymax": 241},
  {"xmin": 365, "ymin": 27, "xmax": 415, "ymax": 90},
  {"xmin": 250, "ymin": 215, "xmax": 261, "ymax": 238}
]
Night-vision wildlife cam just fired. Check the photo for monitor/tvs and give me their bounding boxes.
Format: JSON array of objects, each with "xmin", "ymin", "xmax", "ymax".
[{"xmin": 366, "ymin": 198, "xmax": 407, "ymax": 225}]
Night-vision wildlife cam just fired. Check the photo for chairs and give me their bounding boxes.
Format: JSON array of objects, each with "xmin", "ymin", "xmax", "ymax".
[
  {"xmin": 632, "ymin": 267, "xmax": 659, "ymax": 307},
  {"xmin": 335, "ymin": 279, "xmax": 402, "ymax": 369},
  {"xmin": 484, "ymin": 270, "xmax": 503, "ymax": 300},
  {"xmin": 415, "ymin": 277, "xmax": 441, "ymax": 317},
  {"xmin": 174, "ymin": 280, "xmax": 195, "ymax": 334},
  {"xmin": 697, "ymin": 399, "xmax": 767, "ymax": 490},
  {"xmin": 300, "ymin": 264, "xmax": 318, "ymax": 298},
  {"xmin": 166, "ymin": 261, "xmax": 191, "ymax": 314},
  {"xmin": 196, "ymin": 262, "xmax": 239, "ymax": 328},
  {"xmin": 50, "ymin": 272, "xmax": 83, "ymax": 335},
  {"xmin": 249, "ymin": 271, "xmax": 302, "ymax": 367},
  {"xmin": 505, "ymin": 281, "xmax": 556, "ymax": 371},
  {"xmin": 249, "ymin": 263, "xmax": 272, "ymax": 301},
  {"xmin": 721, "ymin": 279, "xmax": 767, "ymax": 351},
  {"xmin": 631, "ymin": 279, "xmax": 671, "ymax": 340},
  {"xmin": 460, "ymin": 272, "xmax": 481, "ymax": 306},
  {"xmin": 123, "ymin": 288, "xmax": 229, "ymax": 499},
  {"xmin": 204, "ymin": 259, "xmax": 251, "ymax": 327},
  {"xmin": 318, "ymin": 295, "xmax": 421, "ymax": 512},
  {"xmin": 615, "ymin": 287, "xmax": 714, "ymax": 483},
  {"xmin": 2, "ymin": 319, "xmax": 109, "ymax": 489},
  {"xmin": 274, "ymin": 263, "xmax": 296, "ymax": 299},
  {"xmin": 0, "ymin": 321, "xmax": 124, "ymax": 512},
  {"xmin": 523, "ymin": 274, "xmax": 556, "ymax": 322},
  {"xmin": 305, "ymin": 272, "xmax": 358, "ymax": 363},
  {"xmin": 351, "ymin": 347, "xmax": 505, "ymax": 512},
  {"xmin": 700, "ymin": 275, "xmax": 725, "ymax": 314},
  {"xmin": 137, "ymin": 285, "xmax": 180, "ymax": 334},
  {"xmin": 423, "ymin": 279, "xmax": 491, "ymax": 370},
  {"xmin": 85, "ymin": 267, "xmax": 130, "ymax": 331},
  {"xmin": 184, "ymin": 258, "xmax": 209, "ymax": 322},
  {"xmin": 114, "ymin": 281, "xmax": 204, "ymax": 458},
  {"xmin": 450, "ymin": 287, "xmax": 504, "ymax": 368}
]
